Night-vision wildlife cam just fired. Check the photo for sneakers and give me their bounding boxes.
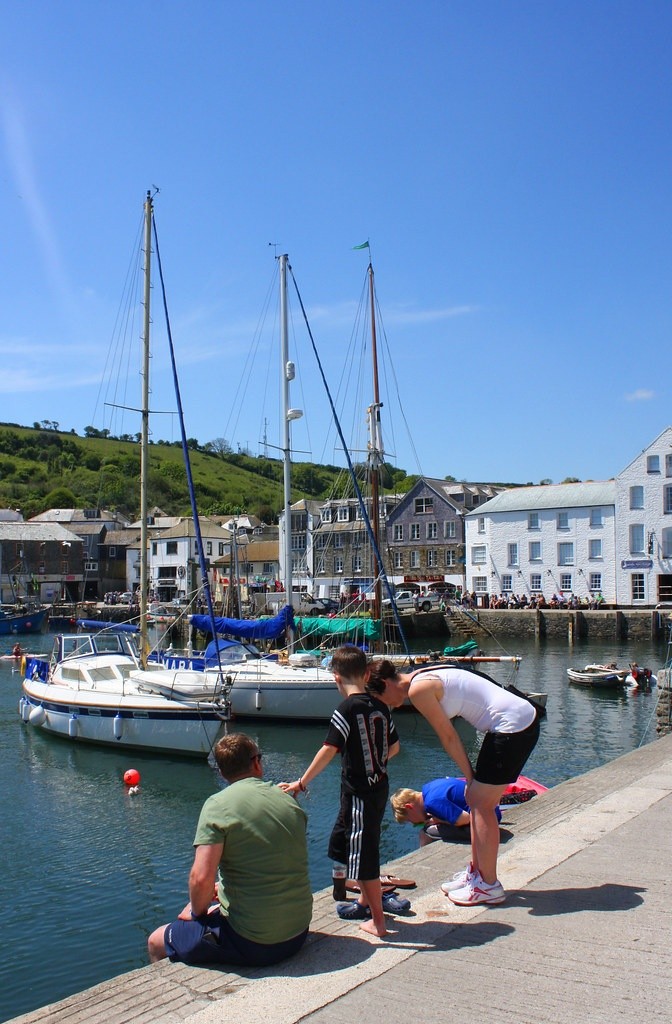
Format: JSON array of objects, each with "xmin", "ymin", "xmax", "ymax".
[
  {"xmin": 448, "ymin": 870, "xmax": 506, "ymax": 906},
  {"xmin": 441, "ymin": 865, "xmax": 483, "ymax": 893}
]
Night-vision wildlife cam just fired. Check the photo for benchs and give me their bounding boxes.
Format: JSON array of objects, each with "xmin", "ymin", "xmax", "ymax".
[{"xmin": 524, "ymin": 603, "xmax": 618, "ymax": 610}]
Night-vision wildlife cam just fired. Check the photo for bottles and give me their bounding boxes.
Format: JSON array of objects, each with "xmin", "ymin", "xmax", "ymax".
[{"xmin": 332, "ymin": 861, "xmax": 347, "ymax": 900}]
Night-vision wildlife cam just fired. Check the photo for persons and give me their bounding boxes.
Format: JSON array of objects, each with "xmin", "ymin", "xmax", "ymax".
[
  {"xmin": 489, "ymin": 593, "xmax": 546, "ymax": 609},
  {"xmin": 551, "ymin": 592, "xmax": 565, "ymax": 610},
  {"xmin": 13, "ymin": 643, "xmax": 28, "ymax": 656},
  {"xmin": 148, "ymin": 734, "xmax": 312, "ymax": 964},
  {"xmin": 277, "ymin": 649, "xmax": 398, "ymax": 937},
  {"xmin": 367, "ymin": 660, "xmax": 541, "ymax": 906},
  {"xmin": 105, "ymin": 591, "xmax": 123, "ymax": 605},
  {"xmin": 413, "ymin": 587, "xmax": 439, "ymax": 611},
  {"xmin": 568, "ymin": 592, "xmax": 604, "ymax": 610},
  {"xmin": 389, "ymin": 777, "xmax": 503, "ymax": 848},
  {"xmin": 444, "ymin": 586, "xmax": 476, "ymax": 609}
]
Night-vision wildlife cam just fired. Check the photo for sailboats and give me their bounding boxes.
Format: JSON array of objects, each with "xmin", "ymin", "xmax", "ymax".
[{"xmin": 18, "ymin": 184, "xmax": 524, "ymax": 763}]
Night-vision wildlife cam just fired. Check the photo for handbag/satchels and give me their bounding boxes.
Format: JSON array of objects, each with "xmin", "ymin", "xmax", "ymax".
[{"xmin": 506, "ymin": 685, "xmax": 547, "ymax": 720}]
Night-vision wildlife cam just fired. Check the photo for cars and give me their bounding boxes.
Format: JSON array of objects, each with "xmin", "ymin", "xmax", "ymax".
[
  {"xmin": 119, "ymin": 592, "xmax": 138, "ymax": 604},
  {"xmin": 315, "ymin": 598, "xmax": 339, "ymax": 614},
  {"xmin": 103, "ymin": 592, "xmax": 120, "ymax": 603}
]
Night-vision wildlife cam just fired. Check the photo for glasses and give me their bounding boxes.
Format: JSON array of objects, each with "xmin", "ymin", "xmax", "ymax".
[{"xmin": 250, "ymin": 754, "xmax": 262, "ymax": 762}]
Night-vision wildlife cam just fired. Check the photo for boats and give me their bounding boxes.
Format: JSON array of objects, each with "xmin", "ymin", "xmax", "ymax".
[
  {"xmin": 566, "ymin": 663, "xmax": 657, "ymax": 688},
  {"xmin": 0, "ymin": 545, "xmax": 54, "ymax": 637},
  {"xmin": 0, "ymin": 653, "xmax": 48, "ymax": 659}
]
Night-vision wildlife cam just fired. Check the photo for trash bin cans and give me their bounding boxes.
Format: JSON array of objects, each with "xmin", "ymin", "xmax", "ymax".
[{"xmin": 477, "ymin": 596, "xmax": 489, "ymax": 609}]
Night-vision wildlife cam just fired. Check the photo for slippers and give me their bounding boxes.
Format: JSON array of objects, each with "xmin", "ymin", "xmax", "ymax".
[
  {"xmin": 379, "ymin": 875, "xmax": 415, "ymax": 888},
  {"xmin": 344, "ymin": 880, "xmax": 396, "ymax": 893},
  {"xmin": 380, "ymin": 893, "xmax": 411, "ymax": 913},
  {"xmin": 337, "ymin": 899, "xmax": 371, "ymax": 919}
]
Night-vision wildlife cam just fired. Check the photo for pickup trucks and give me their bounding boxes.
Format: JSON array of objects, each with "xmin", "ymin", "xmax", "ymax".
[{"xmin": 382, "ymin": 591, "xmax": 440, "ymax": 612}]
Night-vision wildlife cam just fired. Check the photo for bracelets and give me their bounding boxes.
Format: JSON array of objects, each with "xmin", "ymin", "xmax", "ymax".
[
  {"xmin": 299, "ymin": 779, "xmax": 311, "ymax": 800},
  {"xmin": 191, "ymin": 913, "xmax": 199, "ymax": 919}
]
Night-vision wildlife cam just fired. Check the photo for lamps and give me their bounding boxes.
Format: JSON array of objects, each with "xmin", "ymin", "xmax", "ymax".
[
  {"xmin": 547, "ymin": 570, "xmax": 551, "ymax": 576},
  {"xmin": 306, "ymin": 566, "xmax": 311, "ymax": 579},
  {"xmin": 517, "ymin": 570, "xmax": 521, "ymax": 577},
  {"xmin": 577, "ymin": 569, "xmax": 582, "ymax": 575},
  {"xmin": 491, "ymin": 571, "xmax": 495, "ymax": 577},
  {"xmin": 287, "ymin": 409, "xmax": 302, "ymax": 421}
]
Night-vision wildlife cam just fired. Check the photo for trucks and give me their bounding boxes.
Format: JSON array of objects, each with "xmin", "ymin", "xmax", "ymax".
[{"xmin": 254, "ymin": 592, "xmax": 325, "ymax": 616}]
